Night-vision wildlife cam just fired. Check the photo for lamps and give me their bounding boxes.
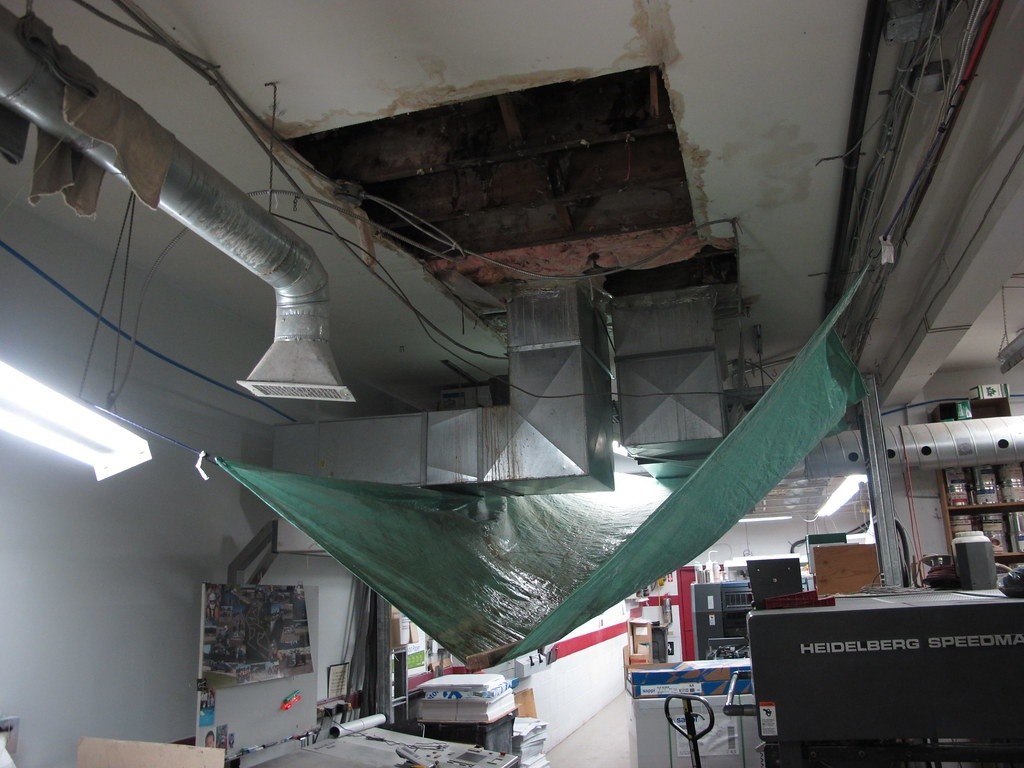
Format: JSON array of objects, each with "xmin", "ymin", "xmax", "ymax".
[
  {"xmin": 997, "ymin": 287, "xmax": 1024, "ymax": 374},
  {"xmin": 0, "ymin": 189, "xmax": 155, "ymax": 482}
]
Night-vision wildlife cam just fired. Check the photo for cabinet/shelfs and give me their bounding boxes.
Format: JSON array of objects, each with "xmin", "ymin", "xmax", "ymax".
[{"xmin": 934, "ymin": 461, "xmax": 1024, "ymax": 564}]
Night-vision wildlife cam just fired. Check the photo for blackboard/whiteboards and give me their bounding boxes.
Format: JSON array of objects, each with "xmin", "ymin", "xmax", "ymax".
[{"xmin": 191, "ymin": 582, "xmax": 321, "ymax": 763}]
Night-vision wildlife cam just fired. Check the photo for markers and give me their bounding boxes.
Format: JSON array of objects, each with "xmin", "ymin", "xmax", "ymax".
[
  {"xmin": 225, "ymin": 735, "xmax": 294, "ymax": 761},
  {"xmin": 281, "ymin": 689, "xmax": 302, "ymax": 709}
]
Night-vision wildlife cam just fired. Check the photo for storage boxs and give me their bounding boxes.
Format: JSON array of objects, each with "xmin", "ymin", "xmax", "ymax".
[
  {"xmin": 928, "ymin": 399, "xmax": 974, "ymax": 421},
  {"xmin": 968, "ymin": 383, "xmax": 1012, "ymax": 400},
  {"xmin": 625, "ymin": 658, "xmax": 754, "ymax": 698}
]
